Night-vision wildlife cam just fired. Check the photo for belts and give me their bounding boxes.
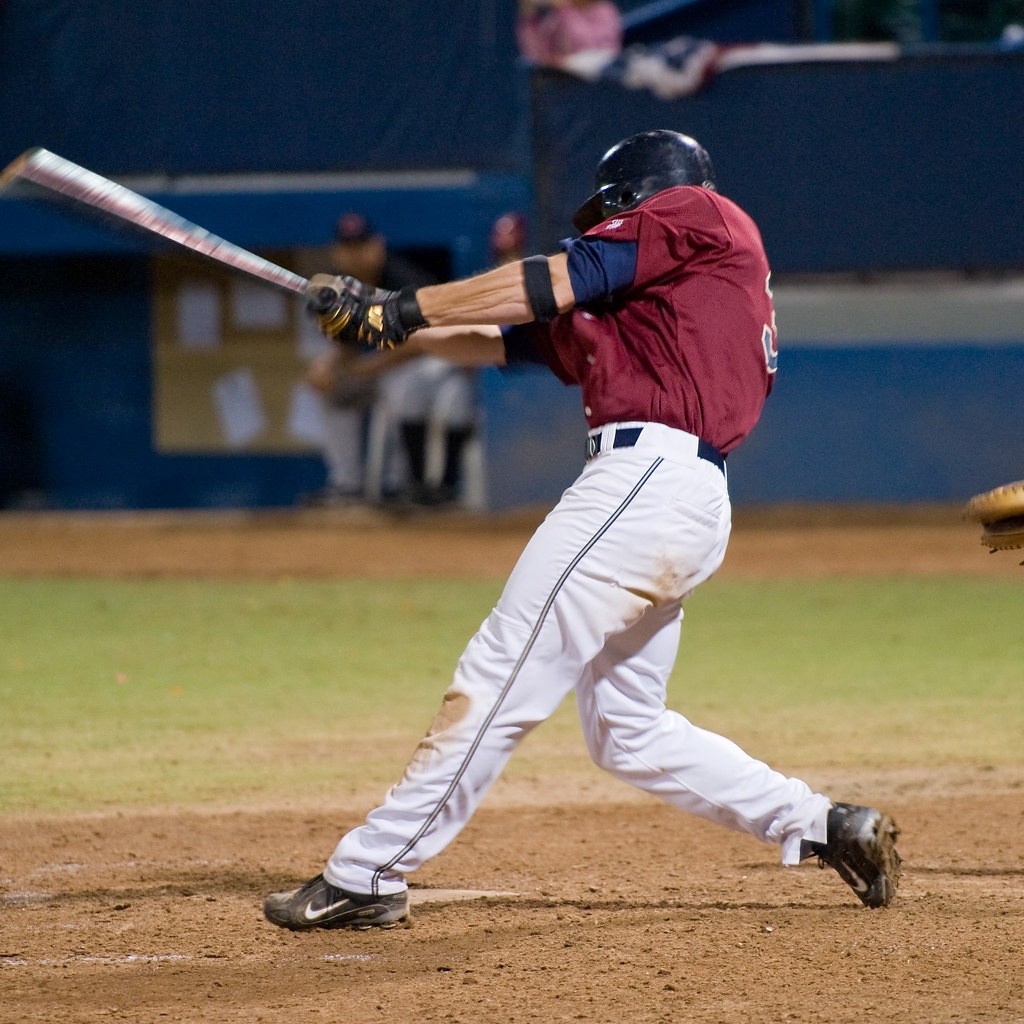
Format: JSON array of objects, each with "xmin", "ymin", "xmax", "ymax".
[{"xmin": 584, "ymin": 428, "xmax": 726, "ymax": 474}]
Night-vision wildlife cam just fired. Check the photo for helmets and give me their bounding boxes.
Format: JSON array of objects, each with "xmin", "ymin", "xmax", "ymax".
[{"xmin": 568, "ymin": 127, "xmax": 722, "ymax": 238}]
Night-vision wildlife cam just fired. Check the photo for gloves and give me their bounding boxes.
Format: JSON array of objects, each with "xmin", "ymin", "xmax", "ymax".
[{"xmin": 310, "ymin": 282, "xmax": 431, "ymax": 350}]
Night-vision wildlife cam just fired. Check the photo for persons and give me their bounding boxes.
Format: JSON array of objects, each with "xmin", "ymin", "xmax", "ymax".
[
  {"xmin": 286, "ymin": 209, "xmax": 535, "ymax": 508},
  {"xmin": 264, "ymin": 131, "xmax": 903, "ymax": 930}
]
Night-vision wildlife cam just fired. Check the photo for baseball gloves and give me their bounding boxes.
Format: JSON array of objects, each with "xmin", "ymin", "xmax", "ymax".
[{"xmin": 962, "ymin": 480, "xmax": 1024, "ymax": 551}]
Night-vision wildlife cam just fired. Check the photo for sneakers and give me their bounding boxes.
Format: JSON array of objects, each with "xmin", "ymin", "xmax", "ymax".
[
  {"xmin": 806, "ymin": 801, "xmax": 905, "ymax": 910},
  {"xmin": 264, "ymin": 869, "xmax": 415, "ymax": 933}
]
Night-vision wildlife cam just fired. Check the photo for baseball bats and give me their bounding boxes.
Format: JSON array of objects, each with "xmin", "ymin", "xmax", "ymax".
[{"xmin": 12, "ymin": 145, "xmax": 323, "ymax": 301}]
